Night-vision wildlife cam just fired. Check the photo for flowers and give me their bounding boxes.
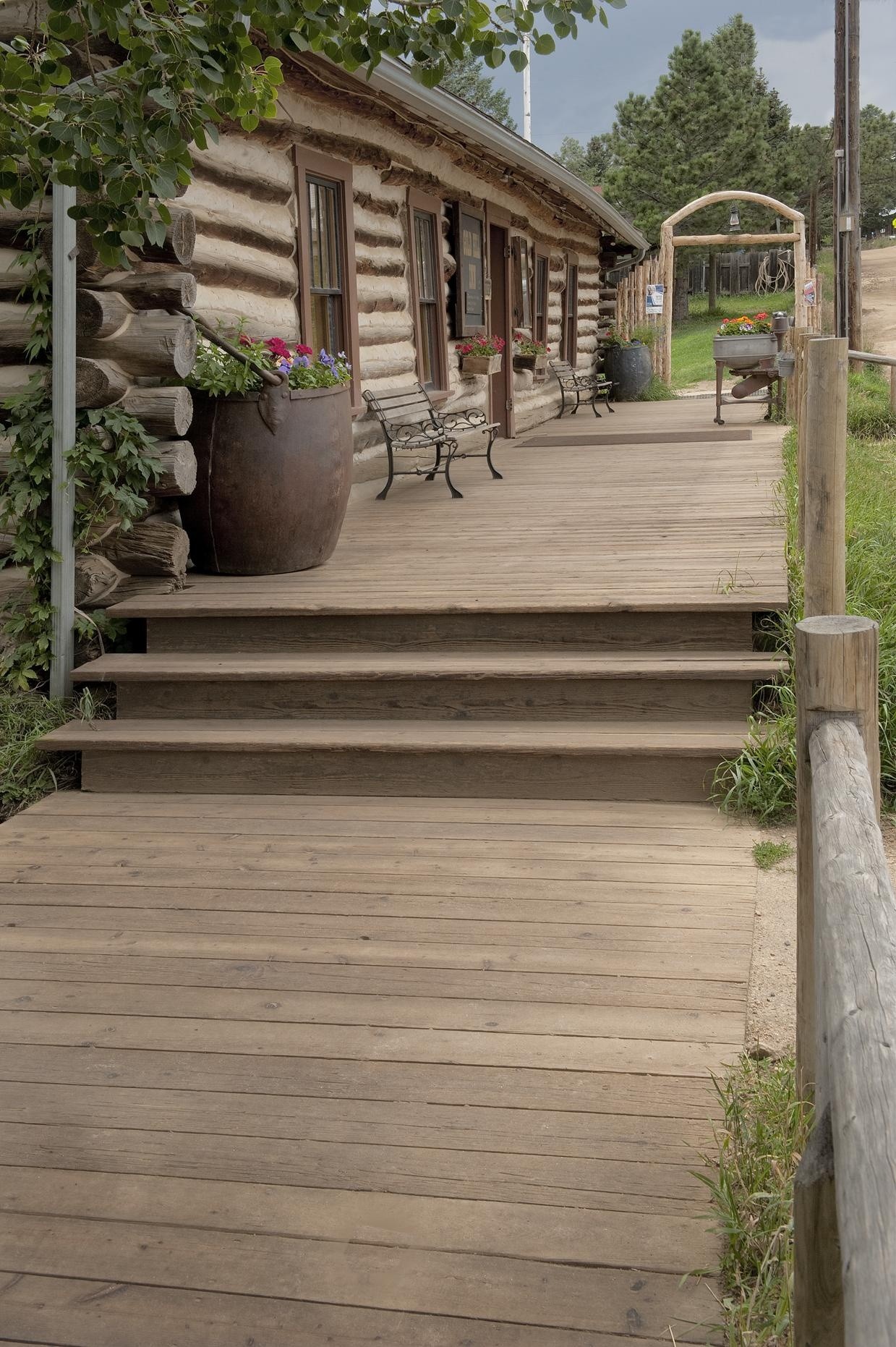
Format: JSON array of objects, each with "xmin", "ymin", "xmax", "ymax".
[
  {"xmin": 189, "ymin": 315, "xmax": 353, "ymax": 398},
  {"xmin": 605, "ymin": 320, "xmax": 653, "ymax": 346},
  {"xmin": 455, "ymin": 334, "xmax": 505, "ymax": 357},
  {"xmin": 513, "ymin": 333, "xmax": 551, "ymax": 355},
  {"xmin": 716, "ymin": 313, "xmax": 772, "ymax": 336}
]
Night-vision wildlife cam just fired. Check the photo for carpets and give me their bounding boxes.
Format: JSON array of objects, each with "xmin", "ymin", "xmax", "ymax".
[{"xmin": 515, "ymin": 429, "xmax": 752, "ymax": 447}]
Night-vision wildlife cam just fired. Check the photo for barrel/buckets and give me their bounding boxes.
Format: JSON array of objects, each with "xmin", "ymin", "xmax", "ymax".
[{"xmin": 779, "ymin": 358, "xmax": 795, "ymax": 377}]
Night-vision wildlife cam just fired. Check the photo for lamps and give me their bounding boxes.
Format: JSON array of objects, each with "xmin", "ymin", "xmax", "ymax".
[{"xmin": 729, "ymin": 203, "xmax": 741, "ymax": 232}]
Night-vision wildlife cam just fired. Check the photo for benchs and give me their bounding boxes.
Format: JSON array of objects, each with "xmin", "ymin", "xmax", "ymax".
[
  {"xmin": 549, "ymin": 360, "xmax": 615, "ymax": 419},
  {"xmin": 362, "ymin": 382, "xmax": 503, "ymax": 500}
]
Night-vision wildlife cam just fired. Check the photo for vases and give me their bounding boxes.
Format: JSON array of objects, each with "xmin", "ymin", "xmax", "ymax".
[
  {"xmin": 604, "ymin": 346, "xmax": 651, "ymax": 402},
  {"xmin": 777, "ymin": 358, "xmax": 795, "ymax": 377},
  {"xmin": 513, "ymin": 354, "xmax": 547, "ymax": 370},
  {"xmin": 462, "ymin": 354, "xmax": 502, "ymax": 375},
  {"xmin": 181, "ymin": 375, "xmax": 353, "ymax": 577},
  {"xmin": 712, "ymin": 334, "xmax": 777, "ymax": 369}
]
